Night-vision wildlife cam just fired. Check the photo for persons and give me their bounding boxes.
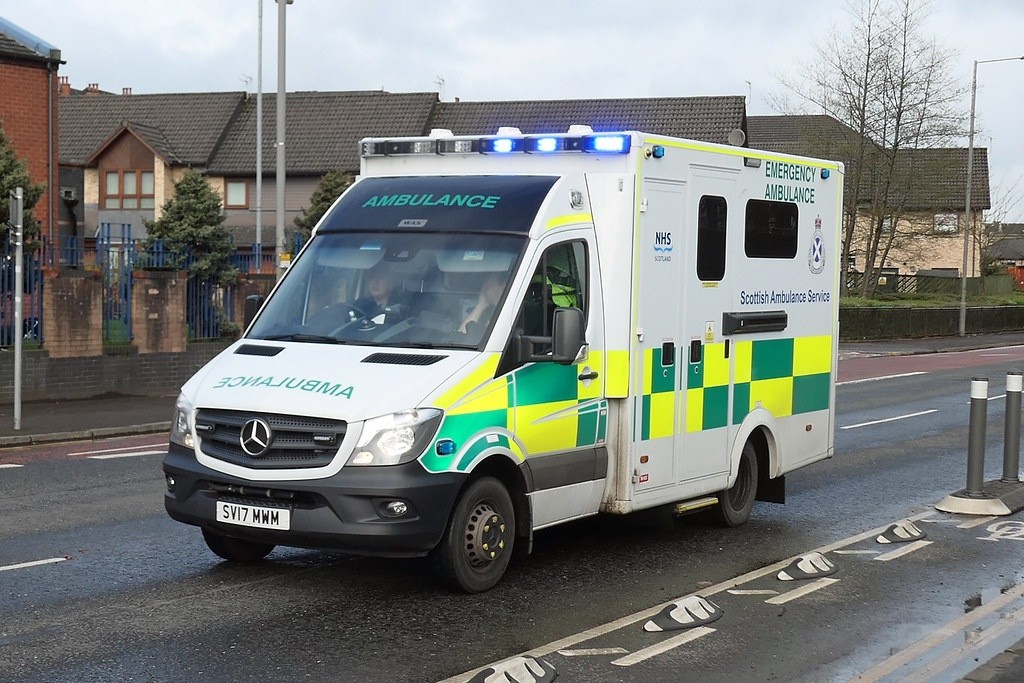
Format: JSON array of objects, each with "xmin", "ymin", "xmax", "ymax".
[
  {"xmin": 353, "ymin": 270, "xmax": 398, "ymax": 318},
  {"xmin": 457, "ymin": 273, "xmax": 527, "ymax": 335}
]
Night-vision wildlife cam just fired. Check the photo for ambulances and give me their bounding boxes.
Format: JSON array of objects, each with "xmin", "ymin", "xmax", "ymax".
[{"xmin": 162, "ymin": 125, "xmax": 845, "ymax": 594}]
